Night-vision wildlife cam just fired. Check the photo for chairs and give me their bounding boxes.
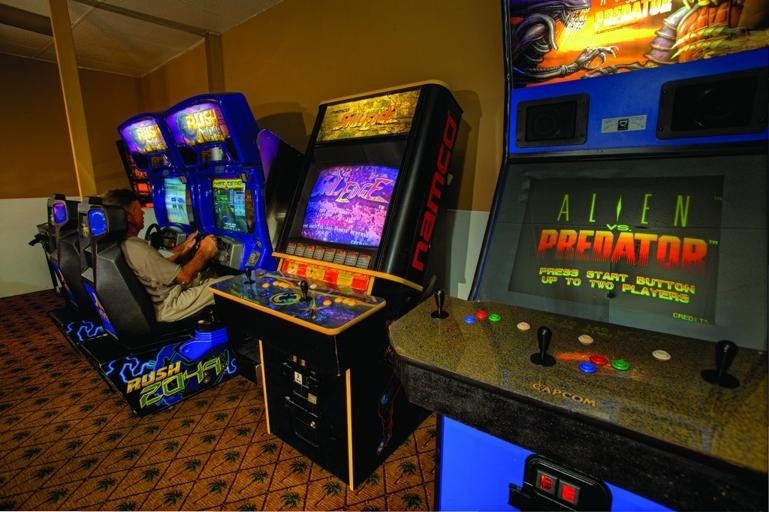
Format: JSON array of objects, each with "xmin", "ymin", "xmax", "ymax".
[{"xmin": 42, "ymin": 191, "xmax": 216, "ymax": 346}]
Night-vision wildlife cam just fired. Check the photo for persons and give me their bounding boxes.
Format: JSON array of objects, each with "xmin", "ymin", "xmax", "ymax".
[{"xmin": 103, "ymin": 188, "xmax": 235, "ymax": 324}]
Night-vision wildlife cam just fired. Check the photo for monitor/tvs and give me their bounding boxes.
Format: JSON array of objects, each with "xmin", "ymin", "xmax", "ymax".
[
  {"xmin": 301, "ymin": 165, "xmax": 399, "ymax": 247},
  {"xmin": 508, "ymin": 175, "xmax": 724, "ymax": 325},
  {"xmin": 212, "ymin": 178, "xmax": 249, "ymax": 233},
  {"xmin": 164, "ymin": 178, "xmax": 189, "ymax": 225}
]
